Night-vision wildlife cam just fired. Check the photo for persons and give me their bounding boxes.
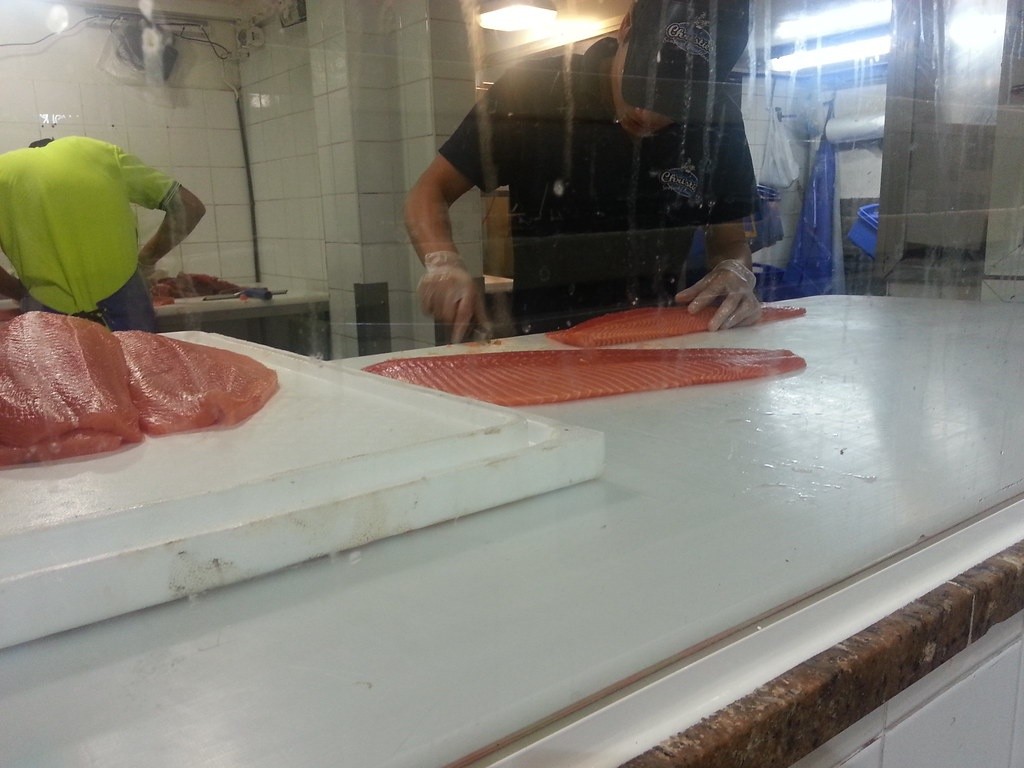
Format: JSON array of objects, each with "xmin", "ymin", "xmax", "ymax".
[
  {"xmin": 1, "ymin": 137, "xmax": 208, "ymax": 335},
  {"xmin": 401, "ymin": 0, "xmax": 766, "ymax": 343}
]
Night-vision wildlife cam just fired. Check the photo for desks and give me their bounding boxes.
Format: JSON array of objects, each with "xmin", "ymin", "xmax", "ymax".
[
  {"xmin": 154, "ymin": 278, "xmax": 328, "ymax": 358},
  {"xmin": 0, "ymin": 293, "xmax": 1024, "ymax": 762}
]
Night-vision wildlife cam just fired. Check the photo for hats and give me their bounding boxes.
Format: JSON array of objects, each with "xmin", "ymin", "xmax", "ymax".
[{"xmin": 621, "ymin": 0, "xmax": 750, "ymax": 123}]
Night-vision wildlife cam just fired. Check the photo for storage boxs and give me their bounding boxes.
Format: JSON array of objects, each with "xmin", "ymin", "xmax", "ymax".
[{"xmin": 846, "ymin": 203, "xmax": 881, "ymax": 259}]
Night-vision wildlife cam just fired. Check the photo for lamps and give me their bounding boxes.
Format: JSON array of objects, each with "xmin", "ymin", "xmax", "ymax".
[
  {"xmin": 476, "ymin": 0, "xmax": 559, "ymax": 34},
  {"xmin": 768, "ymin": 32, "xmax": 891, "ymax": 74}
]
